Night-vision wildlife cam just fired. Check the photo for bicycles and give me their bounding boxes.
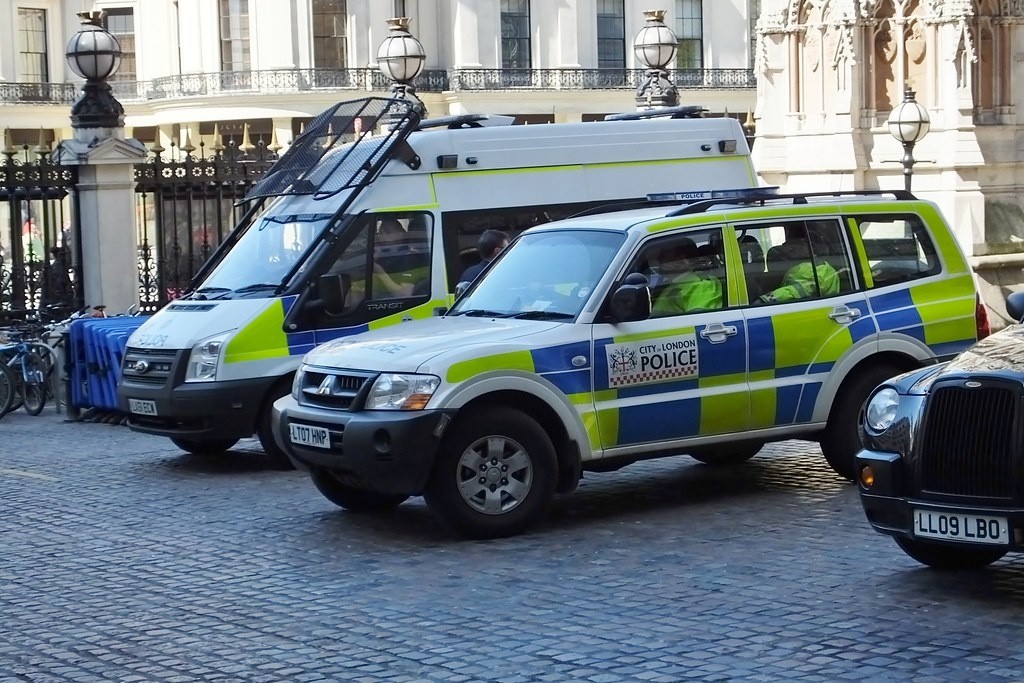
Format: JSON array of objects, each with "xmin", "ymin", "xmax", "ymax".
[{"xmin": 0, "ymin": 304, "xmax": 142, "ymax": 421}]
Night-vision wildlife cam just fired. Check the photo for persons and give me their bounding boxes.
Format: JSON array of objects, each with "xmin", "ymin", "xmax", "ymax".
[
  {"xmin": 37, "ymin": 246, "xmax": 77, "ymax": 321},
  {"xmin": 455, "ymin": 228, "xmax": 510, "ymax": 304},
  {"xmin": 367, "ymin": 219, "xmax": 424, "ymax": 302},
  {"xmin": 757, "ymin": 233, "xmax": 842, "ymax": 304},
  {"xmin": 653, "ymin": 239, "xmax": 721, "ymax": 314},
  {"xmin": 56, "ymin": 221, "xmax": 71, "ymax": 249},
  {"xmin": 22, "ymin": 216, "xmax": 45, "ymax": 261}
]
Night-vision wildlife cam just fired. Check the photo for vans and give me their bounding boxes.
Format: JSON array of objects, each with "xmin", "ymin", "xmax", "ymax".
[{"xmin": 114, "ymin": 103, "xmax": 760, "ymax": 452}]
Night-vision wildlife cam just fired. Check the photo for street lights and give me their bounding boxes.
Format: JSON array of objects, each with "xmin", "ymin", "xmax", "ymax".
[{"xmin": 886, "ymin": 87, "xmax": 930, "ymax": 238}]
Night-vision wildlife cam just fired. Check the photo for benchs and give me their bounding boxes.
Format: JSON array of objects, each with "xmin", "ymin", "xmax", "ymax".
[{"xmin": 739, "ymin": 235, "xmax": 927, "ymax": 292}]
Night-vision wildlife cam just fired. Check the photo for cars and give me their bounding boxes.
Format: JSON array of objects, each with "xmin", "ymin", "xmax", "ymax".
[{"xmin": 854, "ymin": 291, "xmax": 1023, "ymax": 577}]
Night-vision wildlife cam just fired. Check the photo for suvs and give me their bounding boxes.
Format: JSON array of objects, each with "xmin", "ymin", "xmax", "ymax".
[{"xmin": 269, "ymin": 190, "xmax": 991, "ymax": 542}]
